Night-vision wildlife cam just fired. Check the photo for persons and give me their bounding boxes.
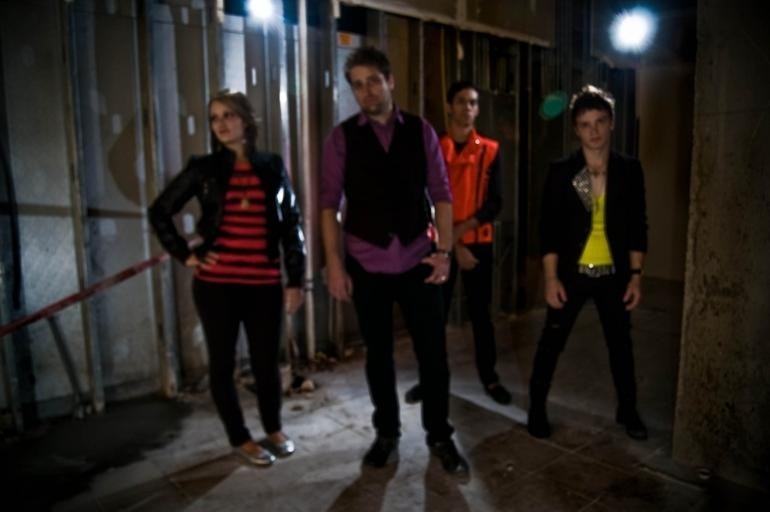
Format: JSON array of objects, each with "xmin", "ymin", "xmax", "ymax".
[
  {"xmin": 319, "ymin": 45, "xmax": 470, "ymax": 475},
  {"xmin": 405, "ymin": 80, "xmax": 512, "ymax": 405},
  {"xmin": 146, "ymin": 87, "xmax": 306, "ymax": 465},
  {"xmin": 527, "ymin": 83, "xmax": 649, "ymax": 440}
]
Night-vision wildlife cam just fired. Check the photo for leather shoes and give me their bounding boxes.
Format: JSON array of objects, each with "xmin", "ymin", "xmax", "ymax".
[
  {"xmin": 428, "ymin": 437, "xmax": 468, "ymax": 474},
  {"xmin": 365, "ymin": 435, "xmax": 397, "ymax": 468},
  {"xmin": 236, "ymin": 432, "xmax": 295, "ymax": 465},
  {"xmin": 486, "ymin": 383, "xmax": 510, "ymax": 404},
  {"xmin": 528, "ymin": 409, "xmax": 549, "ymax": 438},
  {"xmin": 406, "ymin": 384, "xmax": 421, "ymax": 403},
  {"xmin": 617, "ymin": 409, "xmax": 646, "ymax": 439}
]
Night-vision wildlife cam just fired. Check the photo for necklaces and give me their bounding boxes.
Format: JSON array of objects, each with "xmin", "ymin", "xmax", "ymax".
[
  {"xmin": 588, "ymin": 170, "xmax": 605, "ymax": 214},
  {"xmin": 234, "ymin": 166, "xmax": 252, "ymax": 209}
]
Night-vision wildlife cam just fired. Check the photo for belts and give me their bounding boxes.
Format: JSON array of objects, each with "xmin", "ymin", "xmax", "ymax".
[{"xmin": 578, "ymin": 264, "xmax": 614, "ymax": 277}]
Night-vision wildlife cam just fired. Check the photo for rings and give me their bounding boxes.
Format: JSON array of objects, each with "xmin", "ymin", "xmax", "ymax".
[{"xmin": 440, "ymin": 275, "xmax": 447, "ymax": 281}]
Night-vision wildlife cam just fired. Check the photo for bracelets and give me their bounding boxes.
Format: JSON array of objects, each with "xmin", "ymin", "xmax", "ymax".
[
  {"xmin": 462, "ymin": 220, "xmax": 470, "ymax": 229},
  {"xmin": 436, "ymin": 249, "xmax": 452, "ymax": 257},
  {"xmin": 630, "ymin": 269, "xmax": 645, "ymax": 277}
]
